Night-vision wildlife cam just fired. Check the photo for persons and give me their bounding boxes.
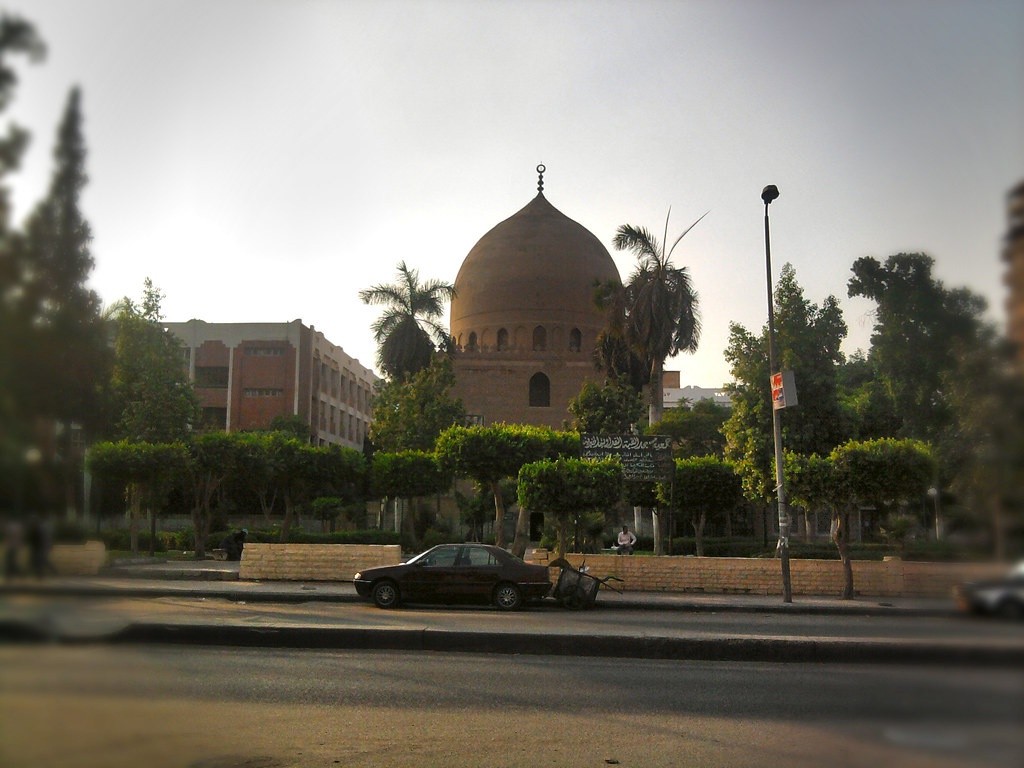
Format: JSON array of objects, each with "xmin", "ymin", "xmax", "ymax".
[
  {"xmin": 220, "ymin": 527, "xmax": 248, "ymax": 561},
  {"xmin": 617, "ymin": 525, "xmax": 637, "ymax": 554}
]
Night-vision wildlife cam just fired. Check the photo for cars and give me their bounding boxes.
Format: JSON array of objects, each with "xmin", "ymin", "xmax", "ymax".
[
  {"xmin": 353, "ymin": 543, "xmax": 553, "ymax": 611},
  {"xmin": 961, "ymin": 562, "xmax": 1024, "ymax": 620}
]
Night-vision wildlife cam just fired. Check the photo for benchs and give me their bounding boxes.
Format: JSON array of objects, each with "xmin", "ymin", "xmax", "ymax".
[
  {"xmin": 602, "ymin": 548, "xmax": 628, "ymax": 555},
  {"xmin": 213, "ymin": 549, "xmax": 229, "ymax": 560}
]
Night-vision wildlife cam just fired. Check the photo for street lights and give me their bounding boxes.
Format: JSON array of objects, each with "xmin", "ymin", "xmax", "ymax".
[{"xmin": 760, "ymin": 183, "xmax": 794, "ymax": 602}]
